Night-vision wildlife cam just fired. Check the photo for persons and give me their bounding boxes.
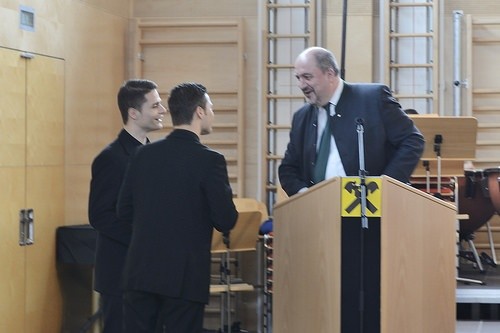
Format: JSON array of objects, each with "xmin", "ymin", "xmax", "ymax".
[
  {"xmin": 278, "ymin": 46, "xmax": 426, "ymax": 199},
  {"xmin": 114, "ymin": 82, "xmax": 239, "ymax": 333},
  {"xmin": 88, "ymin": 78, "xmax": 167, "ymax": 333}
]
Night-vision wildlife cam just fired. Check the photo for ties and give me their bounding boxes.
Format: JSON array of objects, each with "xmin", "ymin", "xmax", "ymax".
[{"xmin": 314, "ymin": 103, "xmax": 332, "ymax": 182}]
